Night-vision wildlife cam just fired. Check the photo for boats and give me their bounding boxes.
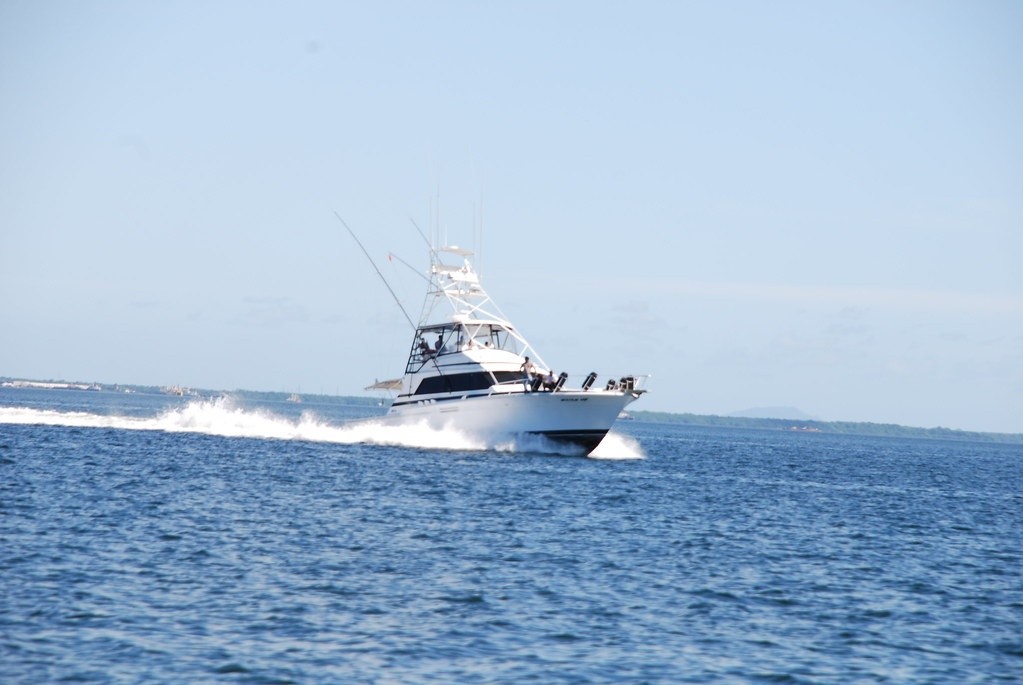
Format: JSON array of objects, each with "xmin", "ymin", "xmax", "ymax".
[{"xmin": 332, "ymin": 207, "xmax": 648, "ymax": 461}]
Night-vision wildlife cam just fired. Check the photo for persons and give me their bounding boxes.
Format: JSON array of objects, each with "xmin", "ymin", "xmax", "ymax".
[
  {"xmin": 543, "ymin": 370, "xmax": 558, "ymax": 392},
  {"xmin": 413, "ymin": 338, "xmax": 431, "ymax": 367},
  {"xmin": 454, "ymin": 335, "xmax": 466, "ymax": 351},
  {"xmin": 485, "ymin": 341, "xmax": 490, "ymax": 349},
  {"xmin": 434, "ymin": 335, "xmax": 446, "ymax": 354},
  {"xmin": 520, "ymin": 356, "xmax": 537, "ymax": 393}
]
